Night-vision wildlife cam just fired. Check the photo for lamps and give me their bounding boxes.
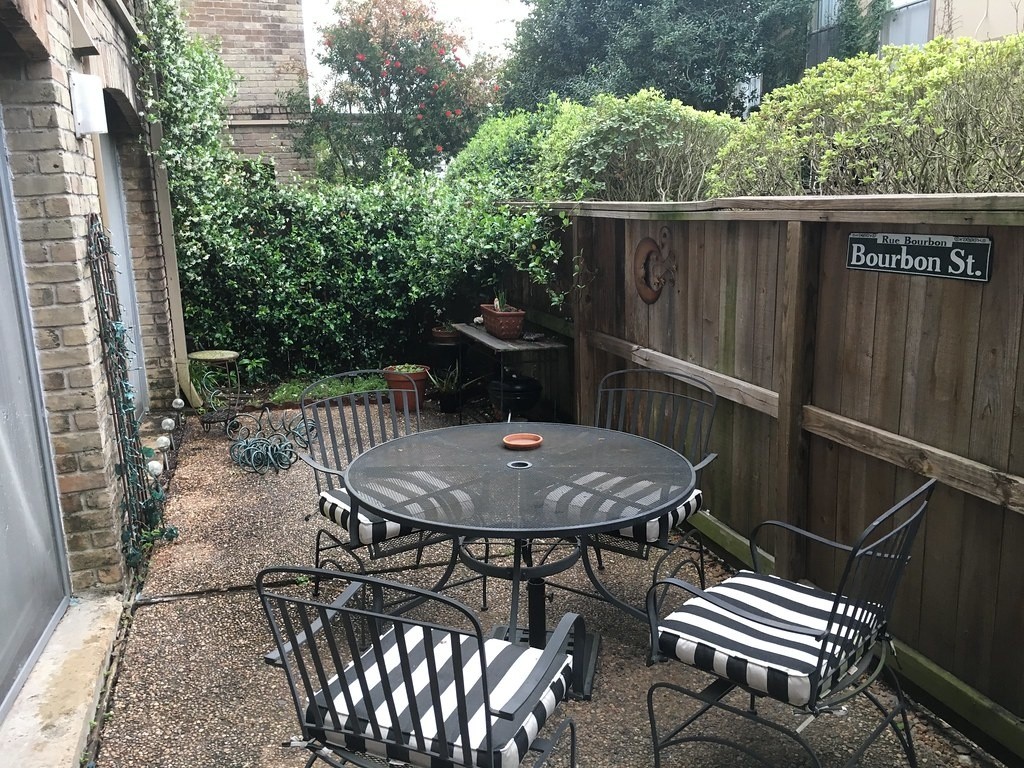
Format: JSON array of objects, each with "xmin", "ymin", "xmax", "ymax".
[
  {"xmin": 171, "ymin": 397, "xmax": 185, "ymax": 431},
  {"xmin": 161, "ymin": 417, "xmax": 176, "ymax": 452},
  {"xmin": 156, "ymin": 436, "xmax": 171, "ymax": 471},
  {"xmin": 146, "ymin": 461, "xmax": 164, "ymax": 493}
]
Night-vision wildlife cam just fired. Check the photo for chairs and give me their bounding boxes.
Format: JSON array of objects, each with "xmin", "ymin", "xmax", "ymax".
[
  {"xmin": 527, "ymin": 366, "xmax": 719, "ymax": 665},
  {"xmin": 646, "ymin": 476, "xmax": 939, "ymax": 768},
  {"xmin": 256, "ymin": 567, "xmax": 603, "ymax": 767},
  {"xmin": 291, "ymin": 368, "xmax": 491, "ymax": 612}
]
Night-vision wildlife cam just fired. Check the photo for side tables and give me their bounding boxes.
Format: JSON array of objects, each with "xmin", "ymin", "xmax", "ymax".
[{"xmin": 187, "ymin": 350, "xmax": 241, "ymax": 431}]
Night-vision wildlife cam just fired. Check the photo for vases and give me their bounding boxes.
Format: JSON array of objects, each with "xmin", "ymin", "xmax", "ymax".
[{"xmin": 433, "ymin": 326, "xmax": 464, "ymax": 364}]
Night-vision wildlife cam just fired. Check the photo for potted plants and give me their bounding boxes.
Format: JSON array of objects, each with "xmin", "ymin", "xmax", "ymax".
[
  {"xmin": 383, "ymin": 363, "xmax": 431, "ymax": 411},
  {"xmin": 426, "ymin": 364, "xmax": 486, "ymax": 413},
  {"xmin": 481, "ymin": 290, "xmax": 527, "ymax": 342}
]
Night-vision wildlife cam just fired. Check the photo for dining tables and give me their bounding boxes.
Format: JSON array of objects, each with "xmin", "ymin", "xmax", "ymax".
[{"xmin": 343, "ymin": 423, "xmax": 701, "ymax": 698}]
